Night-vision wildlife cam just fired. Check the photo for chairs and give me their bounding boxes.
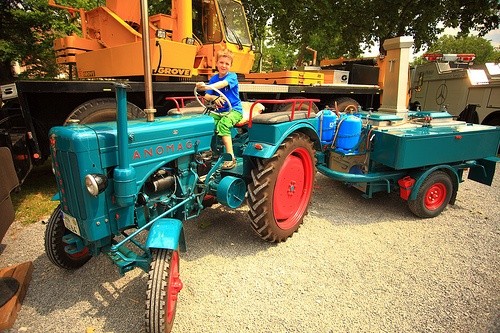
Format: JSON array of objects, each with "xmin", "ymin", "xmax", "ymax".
[{"xmin": 193, "ymin": 13, "xmax": 205, "ymax": 38}]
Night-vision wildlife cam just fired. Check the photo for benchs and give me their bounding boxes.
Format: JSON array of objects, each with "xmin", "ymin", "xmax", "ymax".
[{"xmin": 165, "ymin": 95, "xmax": 321, "ymax": 129}]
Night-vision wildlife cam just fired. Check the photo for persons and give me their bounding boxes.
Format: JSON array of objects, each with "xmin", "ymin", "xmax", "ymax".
[{"xmin": 196, "ymin": 49, "xmax": 242, "ymax": 170}]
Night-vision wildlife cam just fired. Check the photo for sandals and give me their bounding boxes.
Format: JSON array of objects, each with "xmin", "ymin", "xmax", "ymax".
[{"xmin": 221, "ymin": 156, "xmax": 236, "ymax": 169}]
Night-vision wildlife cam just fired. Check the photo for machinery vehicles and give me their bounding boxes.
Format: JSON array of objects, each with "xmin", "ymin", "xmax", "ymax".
[
  {"xmin": 408, "ymin": 53, "xmax": 500, "ymax": 126},
  {"xmin": 0, "ymin": 0, "xmax": 413, "ymax": 173},
  {"xmin": 44, "ymin": 80, "xmax": 500, "ymax": 332}
]
